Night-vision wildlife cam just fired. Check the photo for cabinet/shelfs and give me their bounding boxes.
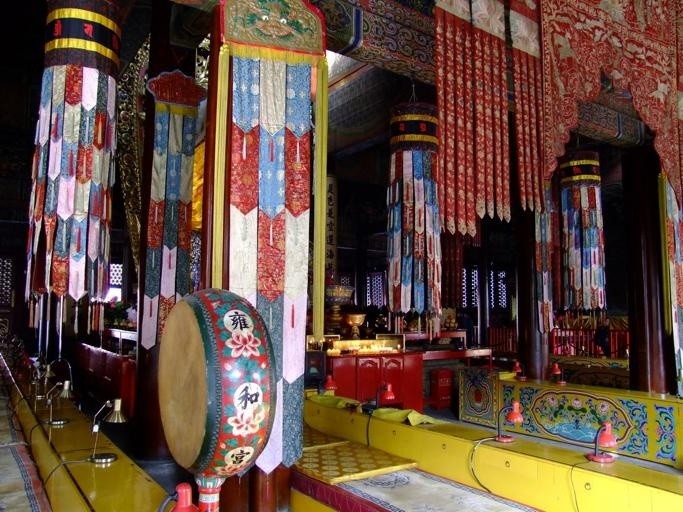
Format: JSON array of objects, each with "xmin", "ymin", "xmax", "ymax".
[{"xmin": 326, "ymin": 352, "xmax": 426, "ymax": 413}]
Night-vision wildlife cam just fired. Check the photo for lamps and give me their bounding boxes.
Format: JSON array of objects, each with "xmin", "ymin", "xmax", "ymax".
[
  {"xmin": 583, "ymin": 422, "xmax": 618, "ymax": 464},
  {"xmin": 513, "ymin": 361, "xmax": 531, "ymax": 384},
  {"xmin": 88, "ymin": 397, "xmax": 127, "ymax": 464},
  {"xmin": 9, "ymin": 354, "xmax": 35, "ymax": 375},
  {"xmin": 595, "ymin": 345, "xmax": 604, "ymax": 359},
  {"xmin": 159, "ymin": 482, "xmax": 198, "ymax": 512},
  {"xmin": 492, "ymin": 400, "xmax": 525, "ymax": 442},
  {"xmin": 317, "ymin": 375, "xmax": 338, "ymax": 397},
  {"xmin": 36, "ymin": 364, "xmax": 53, "ymax": 399},
  {"xmin": 375, "ymin": 382, "xmax": 394, "ymax": 409},
  {"xmin": 44, "ymin": 380, "xmax": 71, "ymax": 426},
  {"xmin": 552, "ymin": 360, "xmax": 566, "ymax": 384}
]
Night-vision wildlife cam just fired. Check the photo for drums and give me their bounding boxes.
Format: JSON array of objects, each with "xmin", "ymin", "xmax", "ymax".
[{"xmin": 158, "ymin": 289, "xmax": 276, "ymax": 512}]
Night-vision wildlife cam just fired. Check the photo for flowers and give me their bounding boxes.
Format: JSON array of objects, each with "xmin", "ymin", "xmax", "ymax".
[{"xmin": 106, "ymin": 297, "xmax": 123, "ymax": 318}]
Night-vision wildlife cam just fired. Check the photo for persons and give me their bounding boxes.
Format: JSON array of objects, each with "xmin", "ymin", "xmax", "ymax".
[{"xmin": 593, "ymin": 317, "xmax": 610, "ymax": 357}]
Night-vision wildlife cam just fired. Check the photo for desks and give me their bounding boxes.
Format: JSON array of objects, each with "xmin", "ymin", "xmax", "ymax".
[
  {"xmin": 0, "ymin": 347, "xmax": 177, "ymax": 512},
  {"xmin": 458, "ymin": 368, "xmax": 516, "ymax": 429},
  {"xmin": 550, "ymin": 354, "xmax": 629, "ymax": 389},
  {"xmin": 416, "ymin": 348, "xmax": 494, "ymax": 370},
  {"xmin": 304, "ymin": 399, "xmax": 683, "ymax": 512},
  {"xmin": 499, "ymin": 378, "xmax": 683, "ymax": 471},
  {"xmin": 400, "ymin": 329, "xmax": 467, "ymax": 348}
]
